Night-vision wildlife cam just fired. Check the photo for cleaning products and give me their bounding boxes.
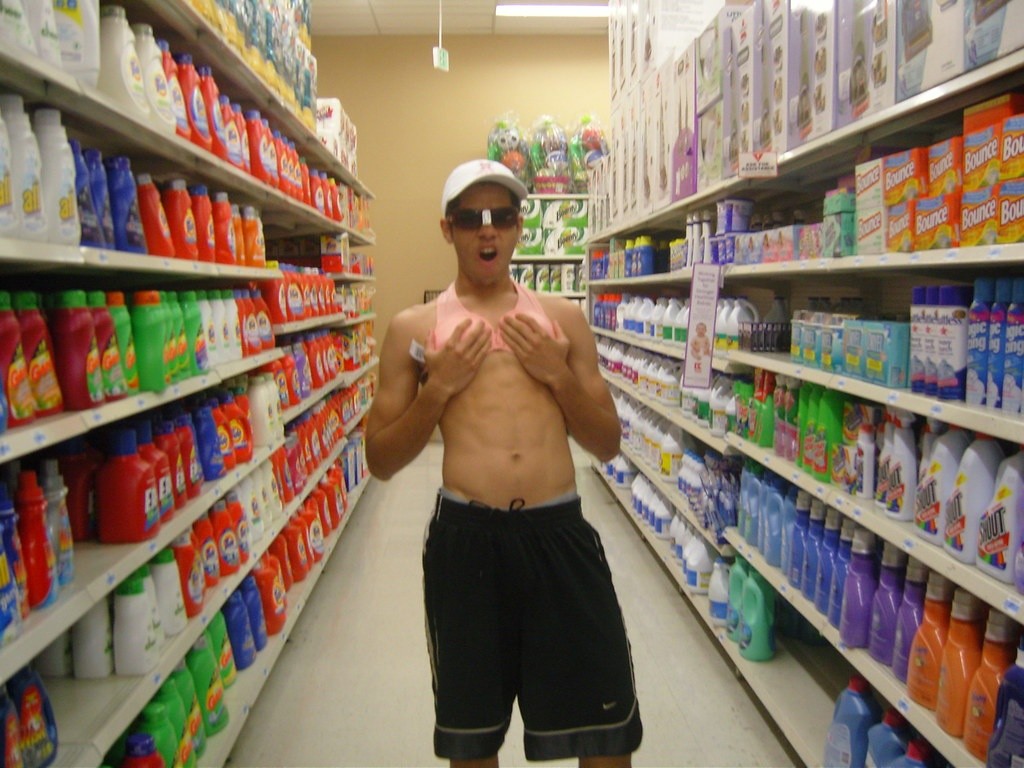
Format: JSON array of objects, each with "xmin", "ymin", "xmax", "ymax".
[
  {"xmin": 508, "ymin": 0, "xmax": 1024, "ymax": 768},
  {"xmin": 0, "ymin": 0, "xmax": 378, "ymax": 768}
]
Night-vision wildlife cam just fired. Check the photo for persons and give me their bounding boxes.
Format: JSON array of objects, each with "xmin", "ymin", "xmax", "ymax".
[{"xmin": 365, "ymin": 158, "xmax": 643, "ymax": 767}]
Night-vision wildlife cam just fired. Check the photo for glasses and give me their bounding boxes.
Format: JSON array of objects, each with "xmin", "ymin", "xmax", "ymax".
[{"xmin": 450, "ymin": 207, "xmax": 518, "ymax": 230}]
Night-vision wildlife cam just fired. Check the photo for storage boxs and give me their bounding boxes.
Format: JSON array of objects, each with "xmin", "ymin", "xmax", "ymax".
[
  {"xmin": 196, "ymin": 0, "xmax": 367, "ymax": 175},
  {"xmin": 584, "ymin": 0, "xmax": 1024, "ymax": 237}
]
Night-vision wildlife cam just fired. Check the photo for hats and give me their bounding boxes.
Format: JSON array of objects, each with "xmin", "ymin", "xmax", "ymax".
[{"xmin": 442, "ymin": 159, "xmax": 528, "ymax": 218}]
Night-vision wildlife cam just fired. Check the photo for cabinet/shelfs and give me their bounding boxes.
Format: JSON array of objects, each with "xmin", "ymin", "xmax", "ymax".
[
  {"xmin": 0, "ymin": 0, "xmax": 383, "ymax": 767},
  {"xmin": 573, "ymin": 51, "xmax": 1024, "ymax": 766},
  {"xmin": 507, "ymin": 190, "xmax": 588, "ymax": 310}
]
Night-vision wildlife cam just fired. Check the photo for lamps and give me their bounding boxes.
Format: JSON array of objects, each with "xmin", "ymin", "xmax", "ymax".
[{"xmin": 495, "ymin": 4, "xmax": 625, "ymax": 19}]
